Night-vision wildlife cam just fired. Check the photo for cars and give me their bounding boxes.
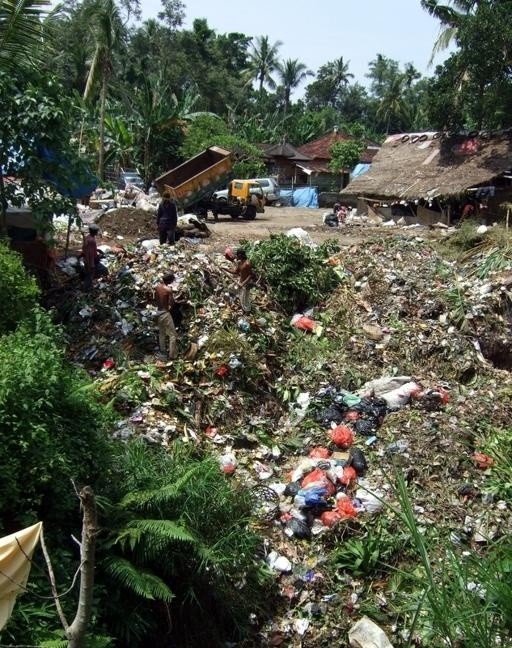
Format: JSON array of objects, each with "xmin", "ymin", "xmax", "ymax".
[
  {"xmin": 118, "ymin": 173, "xmax": 146, "ymax": 191},
  {"xmin": 213, "ymin": 177, "xmax": 281, "ymax": 207}
]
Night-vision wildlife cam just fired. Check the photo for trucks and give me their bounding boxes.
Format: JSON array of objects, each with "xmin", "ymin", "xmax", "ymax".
[{"xmin": 153, "ymin": 145, "xmax": 265, "ymax": 220}]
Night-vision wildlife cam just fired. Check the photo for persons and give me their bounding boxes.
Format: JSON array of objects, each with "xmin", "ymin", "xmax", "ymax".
[
  {"xmin": 153, "ymin": 272, "xmax": 189, "ymax": 360},
  {"xmin": 156, "ymin": 191, "xmax": 177, "ymax": 246},
  {"xmin": 81, "ymin": 225, "xmax": 109, "ymax": 288},
  {"xmin": 221, "ymin": 248, "xmax": 255, "ymax": 314}
]
